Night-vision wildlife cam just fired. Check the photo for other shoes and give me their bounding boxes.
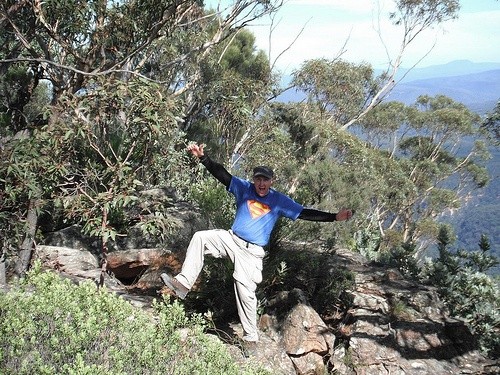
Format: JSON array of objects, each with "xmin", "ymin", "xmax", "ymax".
[
  {"xmin": 242, "ymin": 340, "xmax": 256, "ymax": 356},
  {"xmin": 160, "ymin": 273, "xmax": 189, "ymax": 300}
]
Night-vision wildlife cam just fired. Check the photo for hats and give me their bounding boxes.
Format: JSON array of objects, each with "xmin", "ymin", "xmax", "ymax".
[{"xmin": 254, "ymin": 166, "xmax": 273, "ymax": 179}]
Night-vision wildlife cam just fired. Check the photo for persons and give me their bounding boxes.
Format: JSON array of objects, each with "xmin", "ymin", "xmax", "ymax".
[{"xmin": 160, "ymin": 144, "xmax": 352, "ymax": 343}]
